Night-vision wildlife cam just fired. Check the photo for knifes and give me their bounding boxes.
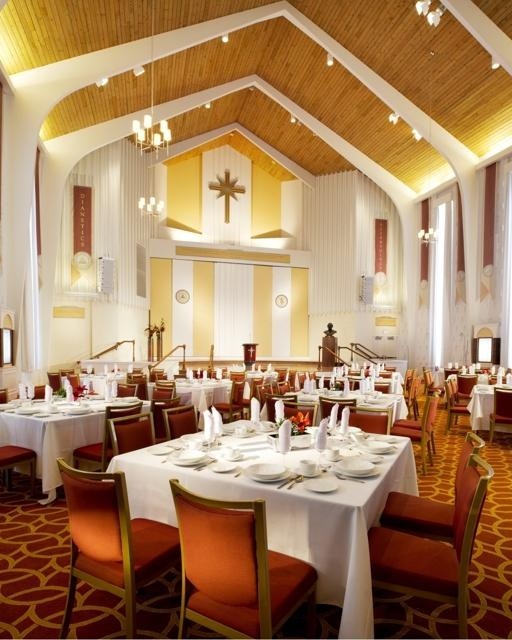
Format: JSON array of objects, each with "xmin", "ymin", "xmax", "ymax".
[{"xmin": 277, "ymin": 475, "xmax": 301, "ymax": 489}]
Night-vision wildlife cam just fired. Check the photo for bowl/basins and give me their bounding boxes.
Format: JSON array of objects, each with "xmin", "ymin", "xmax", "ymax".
[
  {"xmin": 248, "ymin": 462, "xmax": 287, "ymax": 479},
  {"xmin": 170, "ymin": 452, "xmax": 206, "ymax": 463}
]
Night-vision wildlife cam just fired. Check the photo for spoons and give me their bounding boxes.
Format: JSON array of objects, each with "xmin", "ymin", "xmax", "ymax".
[
  {"xmin": 334, "ymin": 473, "xmax": 366, "ymax": 484},
  {"xmin": 289, "ymin": 475, "xmax": 303, "ymax": 490}
]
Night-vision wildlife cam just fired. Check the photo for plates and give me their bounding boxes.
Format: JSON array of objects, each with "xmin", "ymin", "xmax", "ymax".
[
  {"xmin": 173, "ymin": 462, "xmax": 210, "ymax": 467},
  {"xmin": 233, "ymin": 433, "xmax": 253, "ymax": 438},
  {"xmin": 0, "ymin": 402, "xmax": 107, "ymax": 417},
  {"xmin": 259, "ymin": 420, "xmax": 276, "ymax": 432},
  {"xmin": 294, "ymin": 469, "xmax": 322, "ymax": 477},
  {"xmin": 304, "ymin": 478, "xmax": 338, "ymax": 493},
  {"xmin": 245, "ymin": 471, "xmax": 291, "ymax": 483},
  {"xmin": 328, "ymin": 437, "xmax": 394, "ymax": 476},
  {"xmin": 148, "ymin": 445, "xmax": 173, "ymax": 456},
  {"xmin": 212, "ymin": 463, "xmax": 237, "ymax": 473},
  {"xmin": 349, "ymin": 427, "xmax": 360, "ymax": 433}
]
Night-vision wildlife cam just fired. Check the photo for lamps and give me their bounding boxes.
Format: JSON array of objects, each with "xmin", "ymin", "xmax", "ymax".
[
  {"xmin": 417, "ymin": 226, "xmax": 439, "ymax": 248},
  {"xmin": 136, "ymin": 194, "xmax": 169, "ymax": 223},
  {"xmin": 414, "ymin": 0, "xmax": 447, "ymax": 28},
  {"xmin": 131, "ymin": 1, "xmax": 175, "ymax": 159}
]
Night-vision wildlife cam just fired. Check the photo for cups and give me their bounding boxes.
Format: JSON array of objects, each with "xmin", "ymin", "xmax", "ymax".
[
  {"xmin": 300, "ymin": 460, "xmax": 316, "ymax": 474},
  {"xmin": 234, "ymin": 425, "xmax": 250, "ymax": 435}
]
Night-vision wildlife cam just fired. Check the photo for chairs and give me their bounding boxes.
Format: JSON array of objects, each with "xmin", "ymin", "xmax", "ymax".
[
  {"xmin": 368, "ymin": 432, "xmax": 495, "ymax": 640},
  {"xmin": 0, "ymin": 367, "xmax": 439, "ymax": 504},
  {"xmin": 56, "ymin": 457, "xmax": 180, "ymax": 639},
  {"xmin": 423, "ymin": 367, "xmax": 512, "ymax": 442},
  {"xmin": 170, "ymin": 479, "xmax": 318, "ymax": 640}
]
